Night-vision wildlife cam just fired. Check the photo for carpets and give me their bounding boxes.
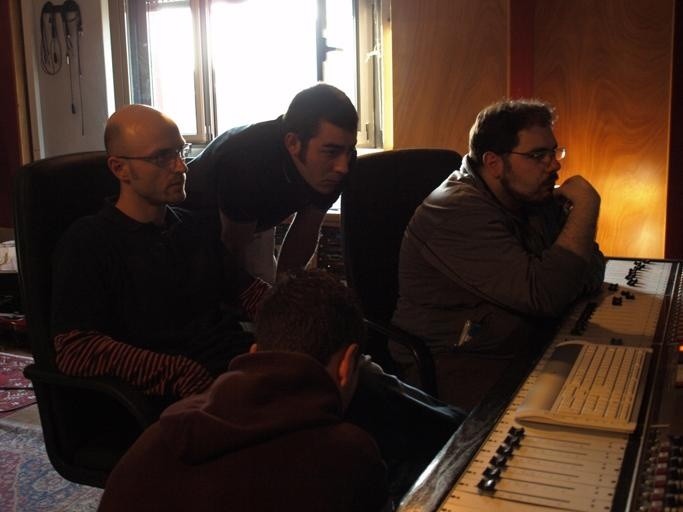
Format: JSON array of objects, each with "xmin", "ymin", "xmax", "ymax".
[
  {"xmin": 0, "ymin": 353, "xmax": 37, "ymax": 419},
  {"xmin": 0, "ymin": 419, "xmax": 105, "ymax": 512}
]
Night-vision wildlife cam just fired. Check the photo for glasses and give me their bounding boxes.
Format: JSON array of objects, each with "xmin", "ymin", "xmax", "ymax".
[
  {"xmin": 507, "ymin": 148, "xmax": 565, "ymax": 164},
  {"xmin": 116, "ymin": 143, "xmax": 191, "ymax": 167}
]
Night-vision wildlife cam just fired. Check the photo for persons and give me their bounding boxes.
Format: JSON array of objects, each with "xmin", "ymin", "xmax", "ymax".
[
  {"xmin": 96, "ymin": 269, "xmax": 392, "ymax": 511},
  {"xmin": 183, "ymin": 83, "xmax": 359, "ymax": 287},
  {"xmin": 383, "ymin": 97, "xmax": 604, "ymax": 414},
  {"xmin": 46, "ymin": 103, "xmax": 270, "ymax": 412}
]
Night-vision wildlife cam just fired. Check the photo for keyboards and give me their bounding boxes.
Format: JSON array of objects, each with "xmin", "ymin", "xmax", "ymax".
[{"xmin": 514, "ymin": 339, "xmax": 653, "ymax": 433}]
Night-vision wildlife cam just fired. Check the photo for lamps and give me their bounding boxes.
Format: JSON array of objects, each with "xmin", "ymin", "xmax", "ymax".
[{"xmin": 0, "ymin": 240, "xmax": 20, "ymax": 311}]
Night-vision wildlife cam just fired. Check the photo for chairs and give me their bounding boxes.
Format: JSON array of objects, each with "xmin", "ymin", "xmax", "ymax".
[
  {"xmin": 345, "ymin": 147, "xmax": 463, "ymax": 395},
  {"xmin": 8, "ymin": 149, "xmax": 262, "ymax": 491}
]
